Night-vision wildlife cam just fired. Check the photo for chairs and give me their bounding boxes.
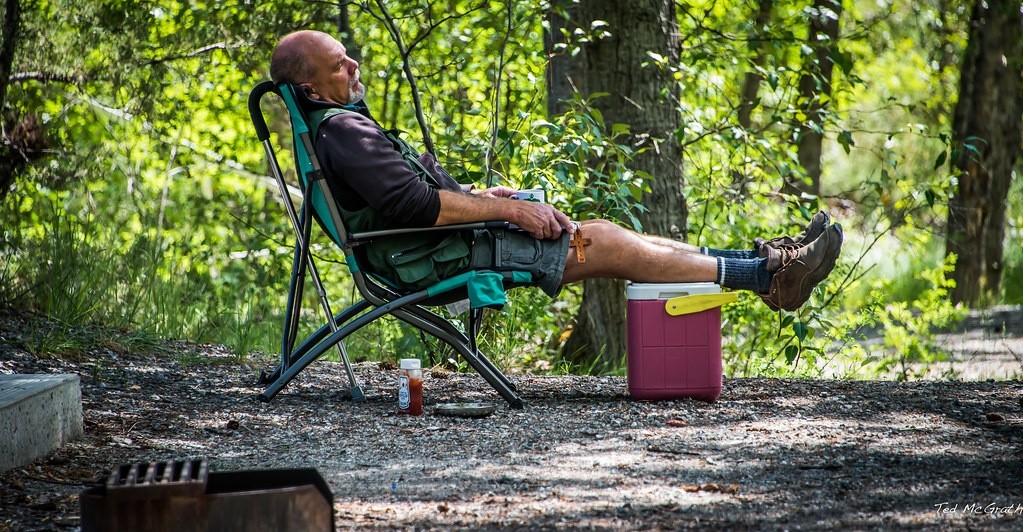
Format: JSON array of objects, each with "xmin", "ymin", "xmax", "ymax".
[{"xmin": 248, "ymin": 80, "xmax": 544, "ymax": 411}]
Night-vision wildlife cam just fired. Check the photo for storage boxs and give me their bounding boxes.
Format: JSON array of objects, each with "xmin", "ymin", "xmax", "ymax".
[{"xmin": 621, "ymin": 279, "xmax": 740, "ymax": 403}]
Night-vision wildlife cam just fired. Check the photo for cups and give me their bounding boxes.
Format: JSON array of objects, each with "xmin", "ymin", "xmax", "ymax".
[{"xmin": 508, "ymin": 190, "xmax": 545, "ymax": 202}]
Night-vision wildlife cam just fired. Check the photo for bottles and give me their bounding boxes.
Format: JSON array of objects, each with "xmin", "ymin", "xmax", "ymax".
[{"xmin": 398, "ymin": 359, "xmax": 423, "ymax": 415}]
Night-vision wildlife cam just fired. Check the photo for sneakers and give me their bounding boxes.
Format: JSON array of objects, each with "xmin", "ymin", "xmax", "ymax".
[{"xmin": 753, "ymin": 207, "xmax": 844, "ymax": 312}]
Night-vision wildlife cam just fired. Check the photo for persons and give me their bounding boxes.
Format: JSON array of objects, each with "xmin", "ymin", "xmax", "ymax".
[{"xmin": 270, "ymin": 31, "xmax": 843, "ymax": 312}]
